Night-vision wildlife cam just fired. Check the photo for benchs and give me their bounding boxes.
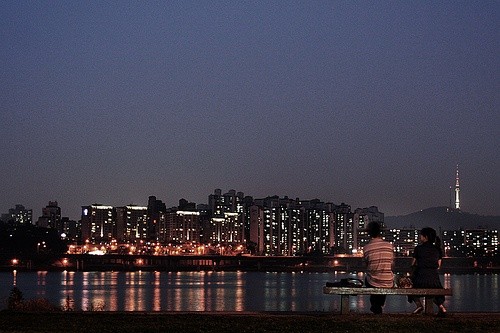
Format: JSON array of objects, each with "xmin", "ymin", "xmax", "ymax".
[{"xmin": 323, "ymin": 279, "xmax": 455, "ymax": 318}]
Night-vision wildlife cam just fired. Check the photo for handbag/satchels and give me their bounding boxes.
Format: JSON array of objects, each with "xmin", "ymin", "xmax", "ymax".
[
  {"xmin": 396, "ymin": 275, "xmax": 412, "ymax": 288},
  {"xmin": 341, "ymin": 278, "xmax": 363, "ymax": 288}
]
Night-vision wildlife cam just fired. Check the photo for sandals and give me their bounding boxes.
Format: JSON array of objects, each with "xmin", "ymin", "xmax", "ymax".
[
  {"xmin": 438, "ymin": 307, "xmax": 447, "ymax": 316},
  {"xmin": 412, "ymin": 306, "xmax": 423, "ymax": 313}
]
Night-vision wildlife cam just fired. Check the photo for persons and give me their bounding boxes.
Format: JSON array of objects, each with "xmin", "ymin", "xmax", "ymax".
[
  {"xmin": 407, "ymin": 226, "xmax": 447, "ymax": 314},
  {"xmin": 363, "ymin": 221, "xmax": 394, "ymax": 314}
]
